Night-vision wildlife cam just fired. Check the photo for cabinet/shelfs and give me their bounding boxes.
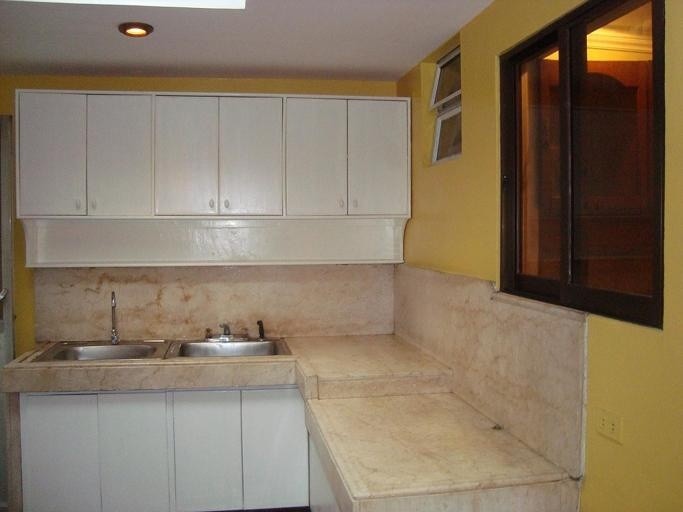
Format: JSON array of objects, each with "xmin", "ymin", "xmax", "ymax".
[
  {"xmin": 15, "ymin": 88, "xmax": 152, "ymax": 218},
  {"xmin": 170, "ymin": 388, "xmax": 312, "ymax": 510},
  {"xmin": 152, "ymin": 93, "xmax": 286, "ymax": 217},
  {"xmin": 287, "ymin": 94, "xmax": 413, "ymax": 217},
  {"xmin": 19, "ymin": 390, "xmax": 169, "ymax": 511}
]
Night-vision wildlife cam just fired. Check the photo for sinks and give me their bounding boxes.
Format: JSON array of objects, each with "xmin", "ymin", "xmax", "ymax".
[
  {"xmin": 31, "ymin": 342, "xmax": 171, "ymax": 361},
  {"xmin": 170, "ymin": 338, "xmax": 291, "ymax": 357}
]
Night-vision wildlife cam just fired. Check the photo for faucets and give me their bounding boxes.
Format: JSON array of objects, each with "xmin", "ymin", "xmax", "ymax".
[
  {"xmin": 220, "ymin": 323, "xmax": 230, "ymax": 334},
  {"xmin": 111, "ymin": 291, "xmax": 116, "ymax": 329},
  {"xmin": 256, "ymin": 320, "xmax": 264, "ymax": 338}
]
